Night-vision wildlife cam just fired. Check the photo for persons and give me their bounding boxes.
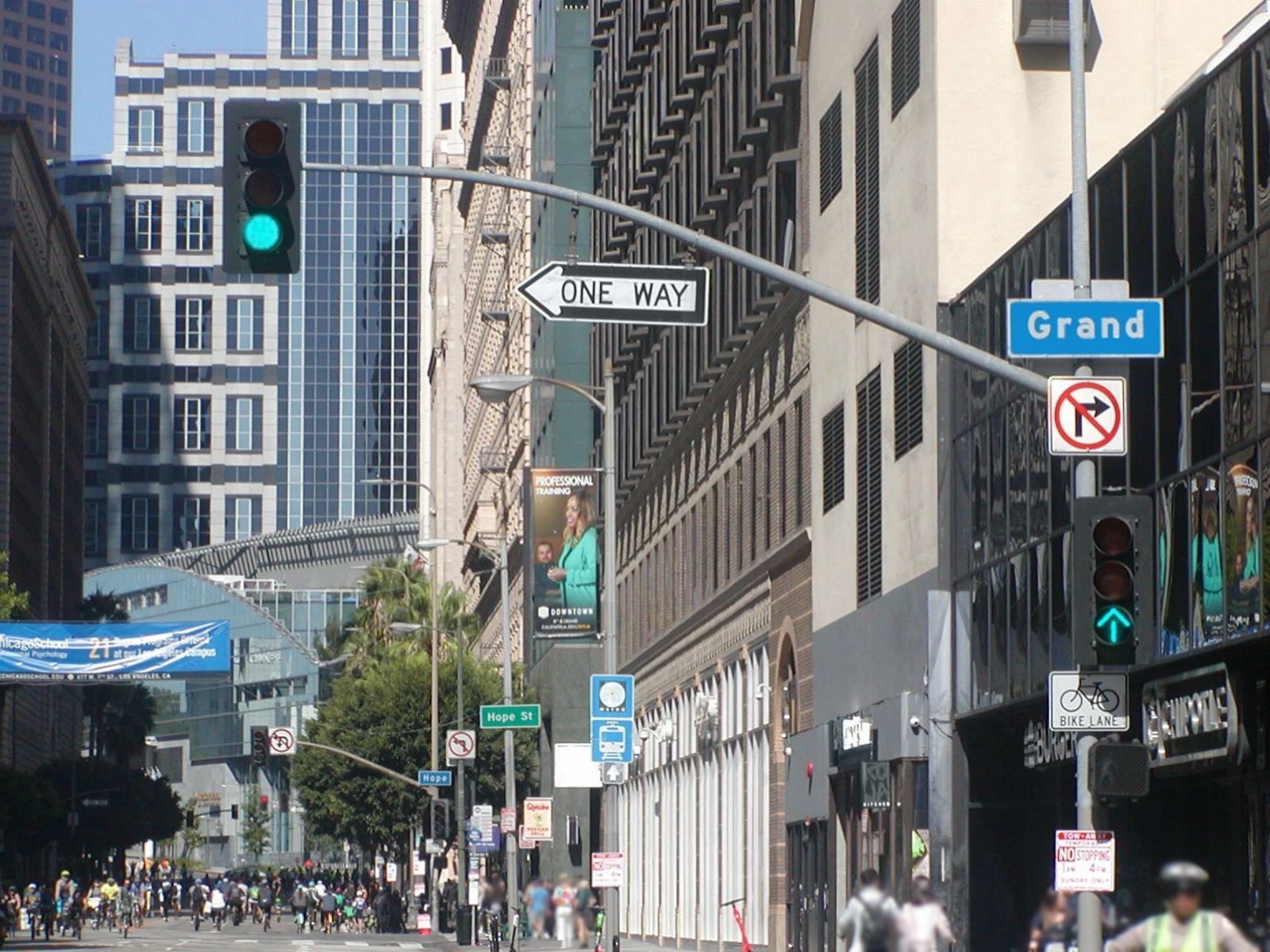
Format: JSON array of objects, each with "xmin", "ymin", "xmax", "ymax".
[
  {"xmin": 1102, "ymin": 860, "xmax": 1262, "ymax": 952},
  {"xmin": 532, "ymin": 490, "xmax": 598, "ymax": 629},
  {"xmin": 1191, "ymin": 497, "xmax": 1260, "ymax": 641},
  {"xmin": 0, "ymin": 857, "xmax": 410, "ymax": 941},
  {"xmin": 836, "ymin": 868, "xmax": 956, "ymax": 952},
  {"xmin": 477, "ymin": 873, "xmax": 597, "ymax": 950},
  {"xmin": 1027, "ymin": 882, "xmax": 1117, "ymax": 952}
]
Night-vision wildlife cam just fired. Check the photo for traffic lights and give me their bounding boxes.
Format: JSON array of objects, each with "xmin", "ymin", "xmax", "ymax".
[
  {"xmin": 250, "ymin": 725, "xmax": 269, "ymax": 766},
  {"xmin": 259, "ymin": 796, "xmax": 268, "ymax": 811},
  {"xmin": 1069, "ymin": 495, "xmax": 1156, "ymax": 666},
  {"xmin": 1088, "ymin": 743, "xmax": 1151, "ymax": 802},
  {"xmin": 220, "ymin": 98, "xmax": 302, "ymax": 273}
]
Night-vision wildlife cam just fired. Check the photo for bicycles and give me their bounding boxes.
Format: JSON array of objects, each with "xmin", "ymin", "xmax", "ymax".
[{"xmin": 0, "ymin": 891, "xmax": 363, "ymax": 944}]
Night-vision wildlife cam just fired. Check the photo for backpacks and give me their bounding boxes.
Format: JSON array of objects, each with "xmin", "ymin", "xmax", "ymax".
[{"xmin": 854, "ymin": 890, "xmax": 899, "ymax": 952}]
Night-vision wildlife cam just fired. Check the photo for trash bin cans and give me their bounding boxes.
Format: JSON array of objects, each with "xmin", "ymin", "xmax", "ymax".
[{"xmin": 456, "ymin": 904, "xmax": 480, "ymax": 944}]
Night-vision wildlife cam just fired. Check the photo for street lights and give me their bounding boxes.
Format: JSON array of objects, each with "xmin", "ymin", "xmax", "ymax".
[
  {"xmin": 358, "ymin": 478, "xmax": 440, "ymax": 933},
  {"xmin": 350, "ymin": 564, "xmax": 410, "ymax": 616},
  {"xmin": 469, "ymin": 357, "xmax": 621, "ymax": 952},
  {"xmin": 412, "ymin": 536, "xmax": 520, "ymax": 952},
  {"xmin": 387, "ymin": 613, "xmax": 467, "ymax": 909}
]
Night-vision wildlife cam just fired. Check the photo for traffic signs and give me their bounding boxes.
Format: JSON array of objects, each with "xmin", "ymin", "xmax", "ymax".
[{"xmin": 513, "ymin": 261, "xmax": 709, "ymax": 327}]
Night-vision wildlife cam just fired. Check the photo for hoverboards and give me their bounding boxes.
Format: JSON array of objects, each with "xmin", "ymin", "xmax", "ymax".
[{"xmin": 719, "ymin": 897, "xmax": 754, "ymax": 952}]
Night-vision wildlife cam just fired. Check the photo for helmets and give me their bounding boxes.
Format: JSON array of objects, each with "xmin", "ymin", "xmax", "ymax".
[
  {"xmin": 28, "ymin": 883, "xmax": 36, "ymax": 889},
  {"xmin": 61, "ymin": 870, "xmax": 70, "ymax": 876},
  {"xmin": 1159, "ymin": 860, "xmax": 1208, "ymax": 884}
]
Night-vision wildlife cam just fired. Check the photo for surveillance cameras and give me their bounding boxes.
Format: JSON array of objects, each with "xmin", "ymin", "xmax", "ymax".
[
  {"xmin": 783, "ymin": 748, "xmax": 792, "ymax": 756},
  {"xmin": 910, "ymin": 718, "xmax": 920, "ymax": 728},
  {"xmin": 640, "ymin": 731, "xmax": 649, "ymax": 742}
]
